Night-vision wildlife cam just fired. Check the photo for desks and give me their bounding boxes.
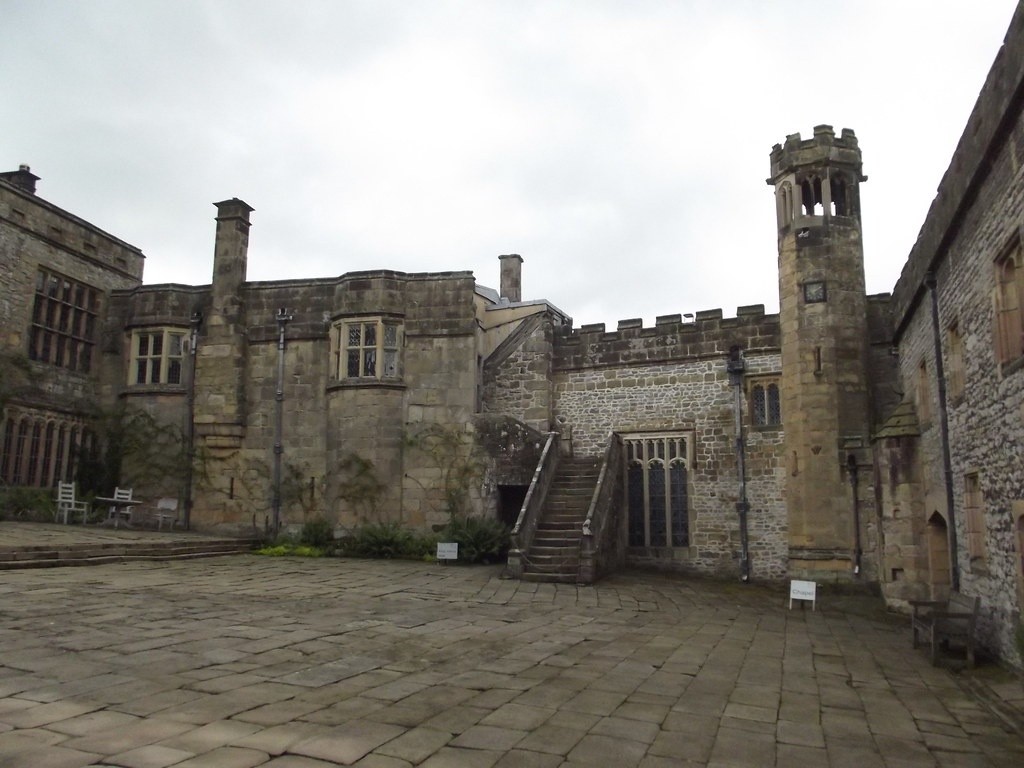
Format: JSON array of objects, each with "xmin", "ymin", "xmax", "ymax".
[{"xmin": 95, "ymin": 496, "xmax": 143, "ymax": 530}]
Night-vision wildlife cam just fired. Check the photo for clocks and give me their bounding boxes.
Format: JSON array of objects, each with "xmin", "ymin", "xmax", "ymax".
[{"xmin": 803, "ymin": 280, "xmax": 827, "ymax": 303}]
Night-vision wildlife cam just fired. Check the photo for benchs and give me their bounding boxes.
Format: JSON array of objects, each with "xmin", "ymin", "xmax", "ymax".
[{"xmin": 908, "ymin": 587, "xmax": 981, "ymax": 668}]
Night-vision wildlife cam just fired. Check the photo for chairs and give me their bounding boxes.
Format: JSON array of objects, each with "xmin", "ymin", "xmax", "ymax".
[
  {"xmin": 143, "ymin": 497, "xmax": 178, "ymax": 532},
  {"xmin": 54, "ymin": 481, "xmax": 88, "ymax": 526},
  {"xmin": 108, "ymin": 487, "xmax": 133, "ymax": 523}
]
